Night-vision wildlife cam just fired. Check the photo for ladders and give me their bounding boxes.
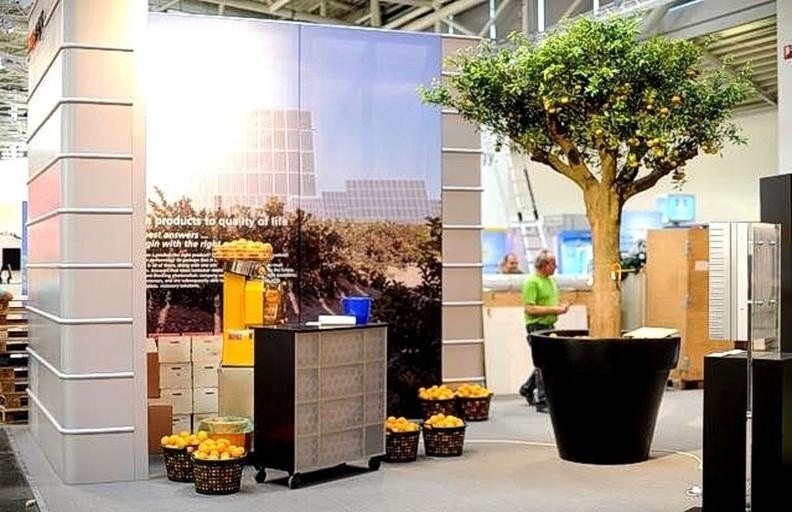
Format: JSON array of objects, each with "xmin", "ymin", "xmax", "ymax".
[{"xmin": 506, "ymin": 146, "xmax": 557, "ymax": 279}]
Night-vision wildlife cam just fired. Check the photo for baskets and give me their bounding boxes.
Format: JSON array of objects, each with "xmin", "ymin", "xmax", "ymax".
[
  {"xmin": 419, "ymin": 422, "xmax": 467, "ymax": 456},
  {"xmin": 159, "ymin": 445, "xmax": 197, "ymax": 482},
  {"xmin": 190, "ymin": 451, "xmax": 248, "ymax": 495},
  {"xmin": 385, "ymin": 426, "xmax": 419, "ymax": 462},
  {"xmin": 419, "ymin": 393, "xmax": 493, "ymax": 421}
]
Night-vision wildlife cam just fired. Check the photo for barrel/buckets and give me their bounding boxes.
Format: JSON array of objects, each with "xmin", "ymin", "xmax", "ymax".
[
  {"xmin": 340, "ymin": 296, "xmax": 372, "ymax": 325},
  {"xmin": 528, "ymin": 328, "xmax": 681, "ymax": 466}
]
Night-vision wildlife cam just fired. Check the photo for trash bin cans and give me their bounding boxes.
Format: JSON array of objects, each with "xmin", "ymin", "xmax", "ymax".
[{"xmin": 201, "ymin": 416, "xmax": 247, "ymax": 451}]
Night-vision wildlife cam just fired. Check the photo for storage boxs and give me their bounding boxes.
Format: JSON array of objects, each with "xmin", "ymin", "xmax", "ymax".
[{"xmin": 156, "ymin": 332, "xmax": 226, "ymax": 433}]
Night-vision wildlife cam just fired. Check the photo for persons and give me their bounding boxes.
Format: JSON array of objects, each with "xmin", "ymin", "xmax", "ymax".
[
  {"xmin": 519, "ymin": 250, "xmax": 570, "ymax": 413},
  {"xmin": 501, "ymin": 254, "xmax": 523, "ymax": 274}
]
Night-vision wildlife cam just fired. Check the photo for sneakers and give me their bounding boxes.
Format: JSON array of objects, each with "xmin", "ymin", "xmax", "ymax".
[
  {"xmin": 536, "ymin": 400, "xmax": 548, "ymax": 411},
  {"xmin": 521, "ymin": 386, "xmax": 536, "ymax": 404}
]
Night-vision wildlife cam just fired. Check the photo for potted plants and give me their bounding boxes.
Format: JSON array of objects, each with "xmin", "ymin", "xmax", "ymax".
[{"xmin": 423, "ymin": 10, "xmax": 757, "ymax": 471}]
